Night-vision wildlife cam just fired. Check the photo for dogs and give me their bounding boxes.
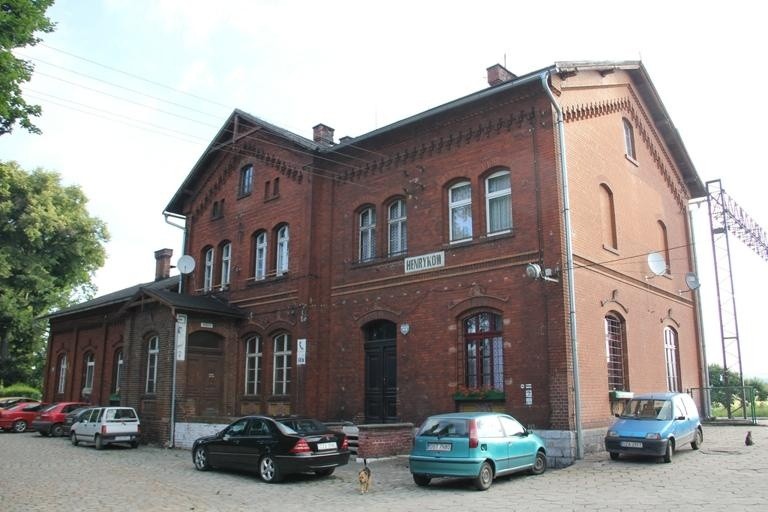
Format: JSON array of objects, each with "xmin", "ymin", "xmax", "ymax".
[
  {"xmin": 745, "ymin": 431, "xmax": 754, "ymax": 446},
  {"xmin": 357, "ymin": 458, "xmax": 371, "ymax": 495}
]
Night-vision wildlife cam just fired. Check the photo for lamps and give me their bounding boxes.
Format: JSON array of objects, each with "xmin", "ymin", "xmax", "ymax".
[{"xmin": 525, "ymin": 263, "xmax": 559, "ymax": 283}]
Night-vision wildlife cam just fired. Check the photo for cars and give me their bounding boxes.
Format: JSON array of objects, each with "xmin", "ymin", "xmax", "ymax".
[
  {"xmin": 190, "ymin": 415, "xmax": 350, "ymax": 483},
  {"xmin": 407, "ymin": 411, "xmax": 548, "ymax": 491},
  {"xmin": 0, "ymin": 395, "xmax": 143, "ymax": 448}
]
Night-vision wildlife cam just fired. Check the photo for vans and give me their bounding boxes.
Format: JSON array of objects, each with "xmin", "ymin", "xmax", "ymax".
[{"xmin": 602, "ymin": 390, "xmax": 703, "ymax": 464}]
